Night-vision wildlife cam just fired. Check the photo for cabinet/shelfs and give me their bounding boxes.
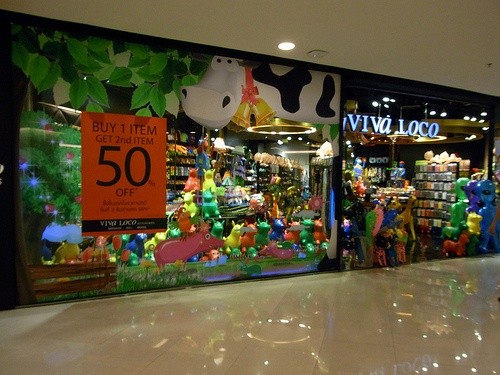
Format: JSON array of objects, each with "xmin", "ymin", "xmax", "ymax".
[
  {"xmin": 162, "ymin": 129, "xmax": 304, "ymax": 237},
  {"xmin": 411, "ymin": 160, "xmax": 472, "ymax": 240}
]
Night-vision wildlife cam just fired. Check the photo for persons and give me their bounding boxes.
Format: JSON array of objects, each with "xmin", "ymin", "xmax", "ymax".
[
  {"xmin": 342, "ymin": 172, "xmax": 352, "ymax": 199},
  {"xmin": 355, "ymin": 176, "xmax": 363, "ymax": 185},
  {"xmin": 489, "ymin": 203, "xmax": 500, "ymax": 234}
]
